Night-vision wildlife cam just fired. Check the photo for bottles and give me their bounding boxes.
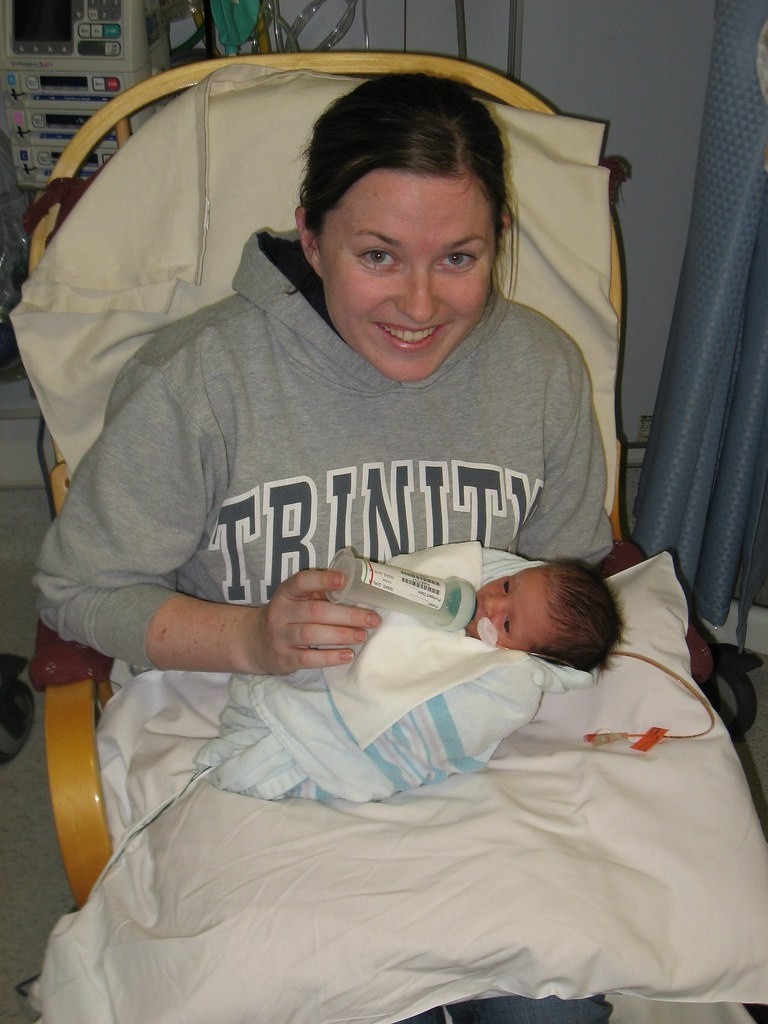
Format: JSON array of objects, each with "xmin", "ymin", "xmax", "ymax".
[{"xmin": 322, "ymin": 548, "xmax": 478, "ymax": 633}]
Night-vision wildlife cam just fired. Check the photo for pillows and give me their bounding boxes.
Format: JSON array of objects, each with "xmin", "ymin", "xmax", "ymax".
[{"xmin": 26, "ymin": 550, "xmax": 768, "ymax": 1024}]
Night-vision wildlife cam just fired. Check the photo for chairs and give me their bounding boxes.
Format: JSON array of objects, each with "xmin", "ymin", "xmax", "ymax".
[{"xmin": 9, "ymin": 52, "xmax": 768, "ymax": 1024}]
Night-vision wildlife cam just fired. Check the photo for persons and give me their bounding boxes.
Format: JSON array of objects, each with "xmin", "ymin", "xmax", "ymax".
[
  {"xmin": 192, "ymin": 540, "xmax": 624, "ymax": 800},
  {"xmin": 33, "ymin": 77, "xmax": 614, "ymax": 1024}
]
